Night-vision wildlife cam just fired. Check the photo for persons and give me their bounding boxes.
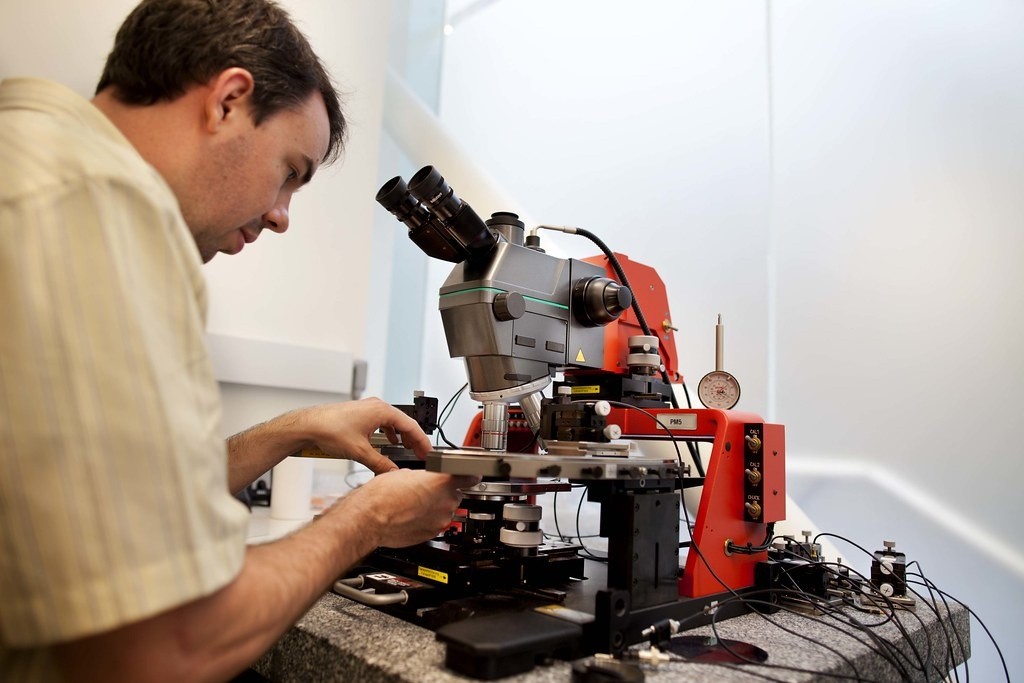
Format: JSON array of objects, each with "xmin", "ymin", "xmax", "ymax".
[{"xmin": 0, "ymin": 0, "xmax": 484, "ymax": 682}]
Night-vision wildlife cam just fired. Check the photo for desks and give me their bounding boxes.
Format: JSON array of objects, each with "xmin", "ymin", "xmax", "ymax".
[{"xmin": 248, "ymin": 578, "xmax": 971, "ymax": 682}]
